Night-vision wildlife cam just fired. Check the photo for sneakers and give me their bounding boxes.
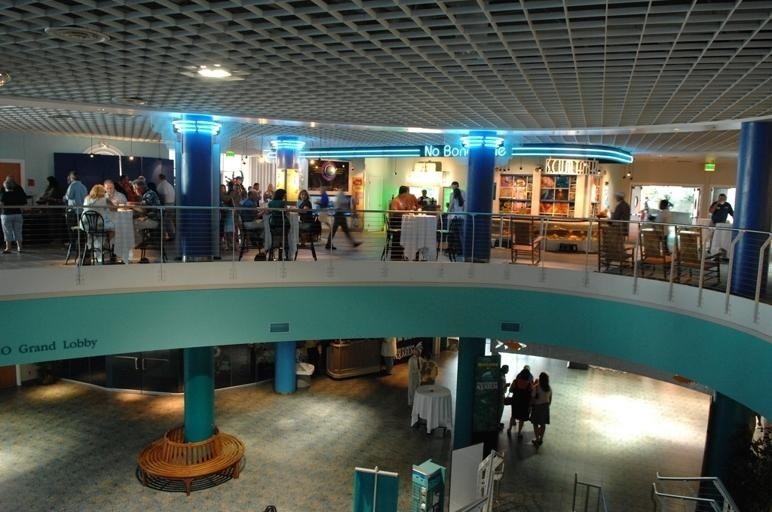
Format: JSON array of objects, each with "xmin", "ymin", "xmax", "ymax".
[
  {"xmin": 518, "ymin": 432, "xmax": 523, "ymax": 437},
  {"xmin": 507, "ymin": 429, "xmax": 511, "ymax": 435},
  {"xmin": 17, "ymin": 247, "xmax": 26, "ymax": 252},
  {"xmin": 2, "ymin": 248, "xmax": 11, "ymax": 254},
  {"xmin": 352, "ymin": 241, "xmax": 362, "ymax": 248},
  {"xmin": 532, "ymin": 439, "xmax": 543, "ymax": 449},
  {"xmin": 325, "ymin": 243, "xmax": 337, "ymax": 250}
]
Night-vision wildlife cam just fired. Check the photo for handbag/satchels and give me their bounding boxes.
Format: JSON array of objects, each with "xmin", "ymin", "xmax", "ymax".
[{"xmin": 503, "ymin": 397, "xmax": 514, "ymax": 406}]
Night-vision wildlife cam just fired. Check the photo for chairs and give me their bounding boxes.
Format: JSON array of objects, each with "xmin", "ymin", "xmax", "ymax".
[
  {"xmin": 376, "ymin": 207, "xmax": 464, "ymax": 265},
  {"xmin": 508, "ymin": 213, "xmax": 541, "ymax": 266},
  {"xmin": 592, "ymin": 217, "xmax": 733, "ymax": 292}
]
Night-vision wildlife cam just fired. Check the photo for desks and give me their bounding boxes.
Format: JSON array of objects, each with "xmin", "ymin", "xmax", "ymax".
[{"xmin": 410, "ymin": 384, "xmax": 452, "ymax": 438}]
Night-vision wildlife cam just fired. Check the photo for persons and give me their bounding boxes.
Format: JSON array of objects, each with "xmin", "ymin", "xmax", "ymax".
[
  {"xmin": 419, "ymin": 350, "xmax": 439, "ymax": 386},
  {"xmin": 36, "ymin": 172, "xmax": 176, "ymax": 267},
  {"xmin": 600, "ymin": 190, "xmax": 632, "ymax": 267},
  {"xmin": 659, "ymin": 193, "xmax": 673, "ymax": 225},
  {"xmin": 508, "ymin": 365, "xmax": 541, "ymax": 441},
  {"xmin": 221, "ymin": 170, "xmax": 364, "ymax": 261},
  {"xmin": 498, "ymin": 364, "xmax": 511, "ymax": 431},
  {"xmin": 381, "ymin": 336, "xmax": 398, "ymax": 377},
  {"xmin": 407, "ymin": 346, "xmax": 427, "ymax": 408},
  {"xmin": 389, "ymin": 181, "xmax": 467, "ymax": 255},
  {"xmin": 0, "ymin": 175, "xmax": 25, "ymax": 254},
  {"xmin": 708, "ymin": 193, "xmax": 734, "ymax": 225},
  {"xmin": 530, "ymin": 371, "xmax": 553, "ymax": 449}
]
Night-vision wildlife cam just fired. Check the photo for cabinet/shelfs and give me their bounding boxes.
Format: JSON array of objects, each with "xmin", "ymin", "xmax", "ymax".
[{"xmin": 499, "ymin": 173, "xmax": 576, "ymax": 218}]
